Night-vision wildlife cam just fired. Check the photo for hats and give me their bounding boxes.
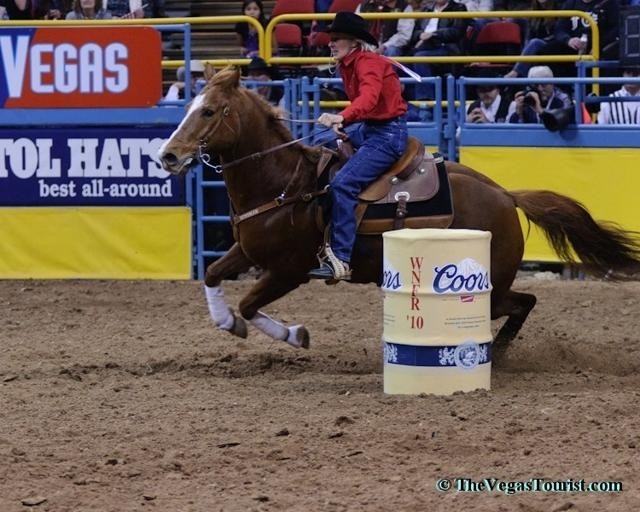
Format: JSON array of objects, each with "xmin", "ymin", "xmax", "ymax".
[
  {"xmin": 473, "ymin": 68, "xmax": 495, "ymax": 77},
  {"xmin": 528, "ymin": 65, "xmax": 553, "ymax": 78},
  {"xmin": 618, "ymin": 60, "xmax": 640, "ymax": 70},
  {"xmin": 241, "ymin": 57, "xmax": 278, "ymax": 76},
  {"xmin": 313, "ymin": 12, "xmax": 379, "ymax": 49}
]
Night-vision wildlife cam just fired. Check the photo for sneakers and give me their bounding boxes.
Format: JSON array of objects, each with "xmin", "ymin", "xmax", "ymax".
[{"xmin": 496, "ymin": 73, "xmax": 509, "ymax": 91}]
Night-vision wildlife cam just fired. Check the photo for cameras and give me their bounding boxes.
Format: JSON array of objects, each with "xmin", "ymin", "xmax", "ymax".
[{"xmin": 518, "ymin": 84, "xmax": 540, "ymax": 106}]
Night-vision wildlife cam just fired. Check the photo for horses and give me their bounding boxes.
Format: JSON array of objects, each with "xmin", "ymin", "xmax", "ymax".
[{"xmin": 156, "ymin": 60, "xmax": 640, "ymax": 365}]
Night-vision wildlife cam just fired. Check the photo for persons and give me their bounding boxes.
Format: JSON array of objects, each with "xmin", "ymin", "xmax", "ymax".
[
  {"xmin": 307, "ymin": 9, "xmax": 409, "ymax": 281},
  {"xmin": 0, "ymin": 0, "xmax": 173, "ymax": 49},
  {"xmin": 164, "ymin": 65, "xmax": 198, "ymax": 107},
  {"xmin": 233, "ymin": 2, "xmax": 639, "ymax": 127}
]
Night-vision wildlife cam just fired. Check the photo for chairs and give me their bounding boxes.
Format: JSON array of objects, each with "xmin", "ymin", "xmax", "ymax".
[{"xmin": 132, "ymin": 0, "xmax": 639, "ymax": 119}]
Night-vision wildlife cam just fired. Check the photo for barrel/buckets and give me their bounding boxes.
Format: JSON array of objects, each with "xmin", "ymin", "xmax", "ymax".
[{"xmin": 383, "ymin": 227, "xmax": 493, "ymax": 395}]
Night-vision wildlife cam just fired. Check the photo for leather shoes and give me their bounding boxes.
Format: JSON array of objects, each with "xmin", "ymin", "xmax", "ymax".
[{"xmin": 308, "ymin": 262, "xmax": 353, "ymax": 281}]
